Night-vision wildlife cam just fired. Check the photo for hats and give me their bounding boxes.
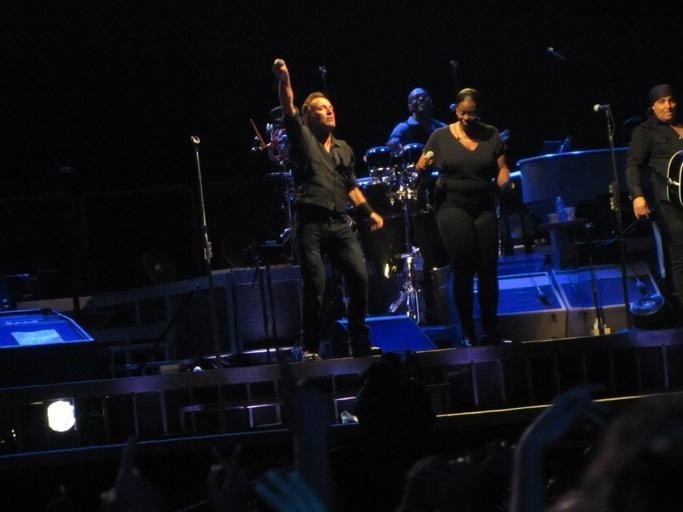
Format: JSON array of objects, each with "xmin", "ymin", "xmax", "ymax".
[{"xmin": 648, "ymin": 84, "xmax": 672, "ymax": 106}]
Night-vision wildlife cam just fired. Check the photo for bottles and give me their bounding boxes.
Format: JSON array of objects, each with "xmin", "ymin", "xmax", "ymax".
[{"xmin": 555, "ymin": 196, "xmax": 568, "ymax": 224}]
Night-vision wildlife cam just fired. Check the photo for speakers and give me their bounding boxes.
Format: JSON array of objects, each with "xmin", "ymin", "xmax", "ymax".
[
  {"xmin": 450, "ymin": 272, "xmax": 566, "ymax": 339},
  {"xmin": 552, "ymin": 258, "xmax": 663, "ymax": 338},
  {"xmin": 504, "ymin": 333, "xmax": 632, "ymax": 408}
]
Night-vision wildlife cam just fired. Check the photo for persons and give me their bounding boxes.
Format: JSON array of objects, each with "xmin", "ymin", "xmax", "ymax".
[
  {"xmin": 266, "ymin": 58, "xmax": 383, "ymax": 360},
  {"xmin": 415, "ymin": 87, "xmax": 514, "ymax": 346},
  {"xmin": 388, "ymin": 88, "xmax": 446, "ymax": 323},
  {"xmin": 352, "ymin": 353, "xmax": 682, "ymax": 511},
  {"xmin": 1, "ymin": 435, "xmax": 327, "ymax": 509},
  {"xmin": 625, "ymin": 85, "xmax": 683, "ymax": 297}
]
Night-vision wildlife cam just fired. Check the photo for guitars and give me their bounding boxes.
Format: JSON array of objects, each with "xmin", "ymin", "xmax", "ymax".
[{"xmin": 666, "ymin": 149, "xmax": 683, "ymax": 209}]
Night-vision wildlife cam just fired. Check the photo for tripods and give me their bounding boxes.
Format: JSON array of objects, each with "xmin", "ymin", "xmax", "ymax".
[
  {"xmin": 190, "ymin": 135, "xmax": 232, "ymax": 370},
  {"xmin": 387, "ymin": 203, "xmax": 432, "ymax": 327}
]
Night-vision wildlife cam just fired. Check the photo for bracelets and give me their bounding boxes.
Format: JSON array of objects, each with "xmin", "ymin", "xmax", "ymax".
[{"xmin": 357, "ymin": 202, "xmax": 373, "ymax": 217}]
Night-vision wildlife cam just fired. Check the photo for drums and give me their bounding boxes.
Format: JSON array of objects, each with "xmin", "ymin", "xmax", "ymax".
[
  {"xmin": 399, "ymin": 143, "xmax": 425, "ymax": 164},
  {"xmin": 356, "ymin": 173, "xmax": 392, "ymax": 219},
  {"xmin": 418, "ymin": 170, "xmax": 441, "ymax": 198},
  {"xmin": 366, "ymin": 145, "xmax": 394, "ymax": 171}
]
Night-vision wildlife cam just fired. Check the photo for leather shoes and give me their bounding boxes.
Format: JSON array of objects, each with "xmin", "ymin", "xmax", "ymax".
[{"xmin": 460, "ymin": 332, "xmax": 498, "ymax": 348}]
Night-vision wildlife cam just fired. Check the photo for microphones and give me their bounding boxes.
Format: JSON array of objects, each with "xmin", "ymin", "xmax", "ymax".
[
  {"xmin": 413, "ymin": 150, "xmax": 436, "ymax": 185},
  {"xmin": 592, "ymin": 103, "xmax": 611, "ymax": 111}
]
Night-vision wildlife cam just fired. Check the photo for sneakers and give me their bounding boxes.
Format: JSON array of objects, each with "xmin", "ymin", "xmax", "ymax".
[
  {"xmin": 352, "ymin": 343, "xmax": 383, "ymax": 359},
  {"xmin": 291, "ymin": 344, "xmax": 320, "ymax": 360}
]
